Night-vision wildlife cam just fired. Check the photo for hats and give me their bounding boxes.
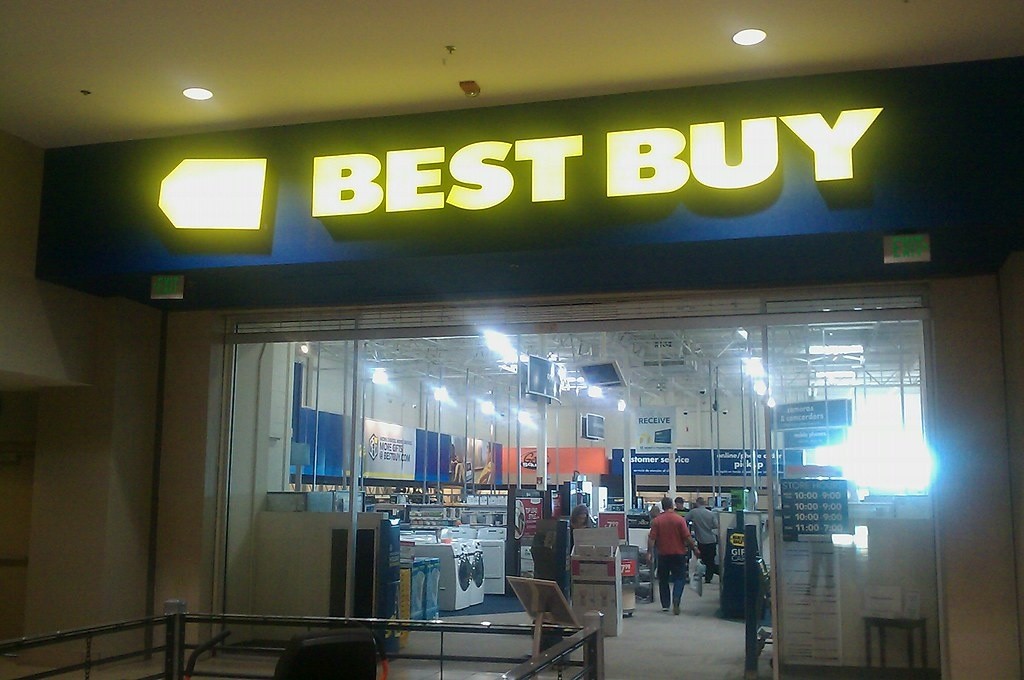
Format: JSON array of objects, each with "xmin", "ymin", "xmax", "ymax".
[{"xmin": 675, "ymin": 497, "xmax": 685, "ymax": 504}]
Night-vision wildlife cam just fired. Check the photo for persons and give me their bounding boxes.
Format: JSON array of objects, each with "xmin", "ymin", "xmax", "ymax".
[
  {"xmin": 571, "ymin": 505, "xmax": 590, "ymax": 529},
  {"xmin": 673, "ymin": 497, "xmax": 690, "ymax": 517},
  {"xmin": 648, "ymin": 497, "xmax": 701, "ymax": 615},
  {"xmin": 684, "ymin": 497, "xmax": 719, "ymax": 583}
]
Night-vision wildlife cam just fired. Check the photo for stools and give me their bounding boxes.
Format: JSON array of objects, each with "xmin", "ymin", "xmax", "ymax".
[{"xmin": 862, "ymin": 611, "xmax": 929, "ymax": 669}]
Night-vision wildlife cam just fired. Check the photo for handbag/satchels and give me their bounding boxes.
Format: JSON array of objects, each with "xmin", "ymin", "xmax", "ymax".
[{"xmin": 690, "ymin": 564, "xmax": 705, "ymax": 597}]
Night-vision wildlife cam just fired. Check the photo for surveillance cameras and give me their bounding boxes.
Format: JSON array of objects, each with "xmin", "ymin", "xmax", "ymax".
[
  {"xmin": 723, "ymin": 412, "xmax": 727, "ymax": 414},
  {"xmin": 413, "ymin": 404, "xmax": 417, "ymax": 408},
  {"xmin": 501, "ymin": 413, "xmax": 505, "ymax": 417},
  {"xmin": 683, "ymin": 411, "xmax": 688, "ymax": 416},
  {"xmin": 699, "ymin": 390, "xmax": 705, "ymax": 394}
]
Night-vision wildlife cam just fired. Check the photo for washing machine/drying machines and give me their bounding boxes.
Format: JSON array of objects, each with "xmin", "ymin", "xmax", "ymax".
[{"xmin": 414, "ymin": 539, "xmax": 486, "ymax": 610}]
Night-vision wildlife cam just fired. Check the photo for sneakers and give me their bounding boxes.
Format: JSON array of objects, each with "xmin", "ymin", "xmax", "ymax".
[
  {"xmin": 674, "ymin": 598, "xmax": 681, "ymax": 615},
  {"xmin": 662, "ymin": 606, "xmax": 670, "ymax": 611}
]
